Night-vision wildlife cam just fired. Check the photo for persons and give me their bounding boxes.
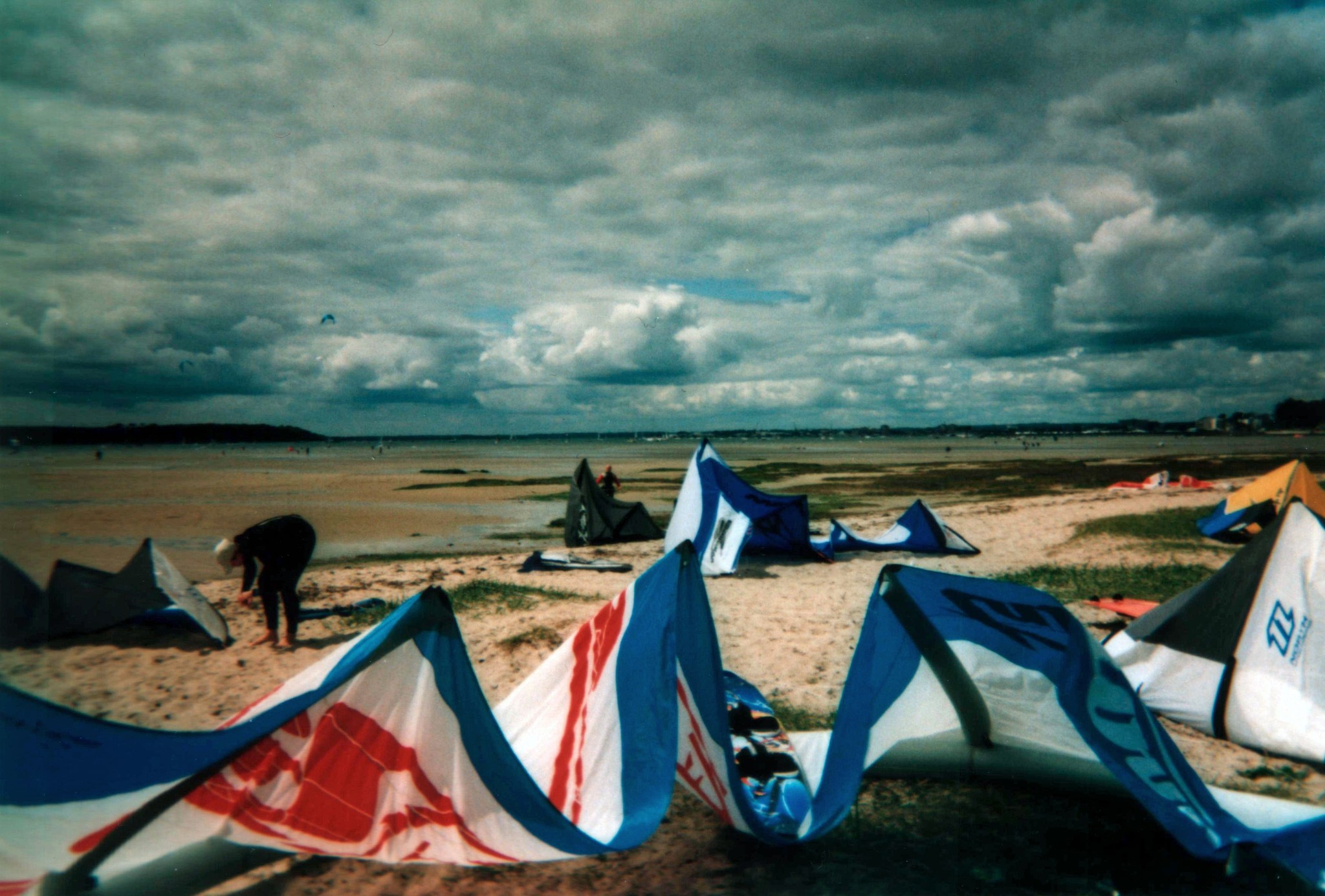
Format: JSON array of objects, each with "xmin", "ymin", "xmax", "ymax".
[
  {"xmin": 216, "ymin": 512, "xmax": 316, "ymax": 655},
  {"xmin": 597, "ymin": 464, "xmax": 623, "ymax": 495}
]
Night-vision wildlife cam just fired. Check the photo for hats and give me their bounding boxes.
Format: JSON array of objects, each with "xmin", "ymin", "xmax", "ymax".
[
  {"xmin": 214, "ymin": 538, "xmax": 235, "ymax": 575},
  {"xmin": 605, "ymin": 465, "xmax": 612, "ymax": 472}
]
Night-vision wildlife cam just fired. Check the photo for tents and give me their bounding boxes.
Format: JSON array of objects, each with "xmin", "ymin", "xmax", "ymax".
[
  {"xmin": 1194, "ymin": 458, "xmax": 1325, "ymax": 544},
  {"xmin": 1100, "ymin": 495, "xmax": 1325, "ymax": 766}
]
